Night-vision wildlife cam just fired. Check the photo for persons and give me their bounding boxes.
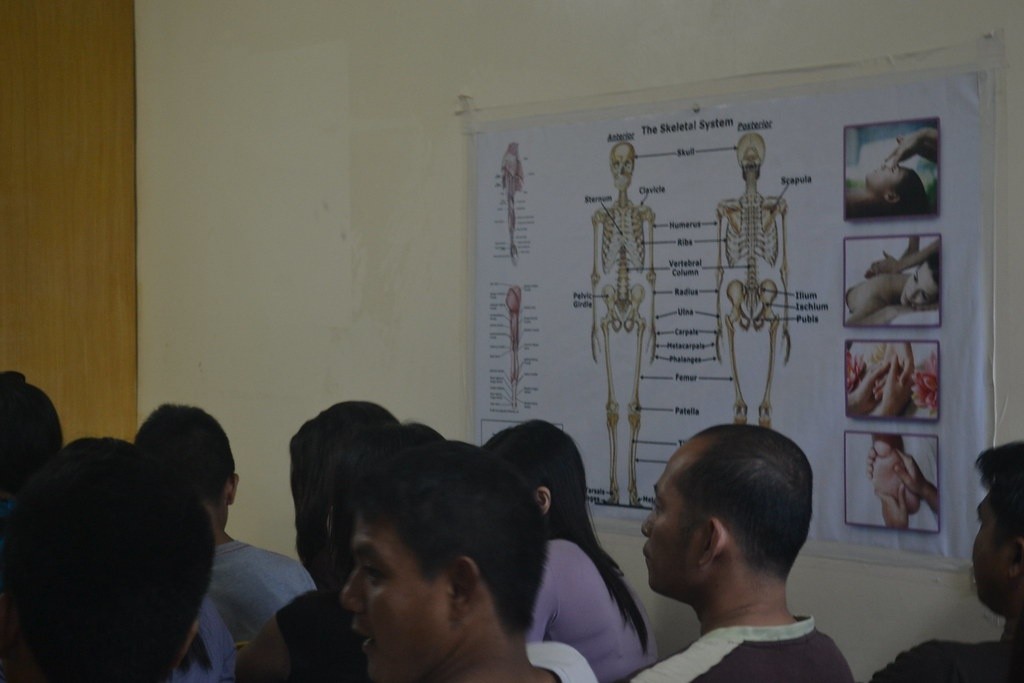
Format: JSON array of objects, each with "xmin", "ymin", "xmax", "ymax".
[
  {"xmin": 845, "ymin": 127, "xmax": 941, "ymax": 530},
  {"xmin": 0, "ymin": 369, "xmax": 1024, "ymax": 683}
]
